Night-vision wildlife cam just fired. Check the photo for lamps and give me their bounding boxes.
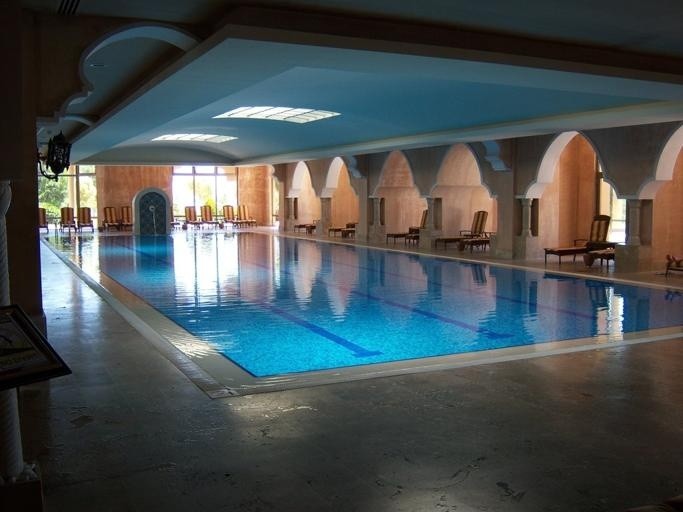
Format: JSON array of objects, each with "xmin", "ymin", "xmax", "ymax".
[{"xmin": 37, "ymin": 130, "xmax": 73, "ymax": 181}]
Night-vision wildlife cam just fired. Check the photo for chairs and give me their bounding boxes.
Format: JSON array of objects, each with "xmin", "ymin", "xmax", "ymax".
[
  {"xmin": 293, "ymin": 219, "xmax": 318, "ymax": 234},
  {"xmin": 329, "ymin": 220, "xmax": 358, "ymax": 237},
  {"xmin": 386, "ymin": 210, "xmax": 427, "ymax": 245},
  {"xmin": 435, "ymin": 211, "xmax": 496, "ymax": 253},
  {"xmin": 170, "ymin": 204, "xmax": 257, "ymax": 230},
  {"xmin": 38, "ymin": 206, "xmax": 134, "ymax": 234},
  {"xmin": 543, "ymin": 213, "xmax": 617, "ymax": 268}
]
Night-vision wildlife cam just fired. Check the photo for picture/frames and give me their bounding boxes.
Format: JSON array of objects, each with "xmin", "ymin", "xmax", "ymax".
[{"xmin": 0, "ymin": 303, "xmax": 72, "ymax": 392}]
